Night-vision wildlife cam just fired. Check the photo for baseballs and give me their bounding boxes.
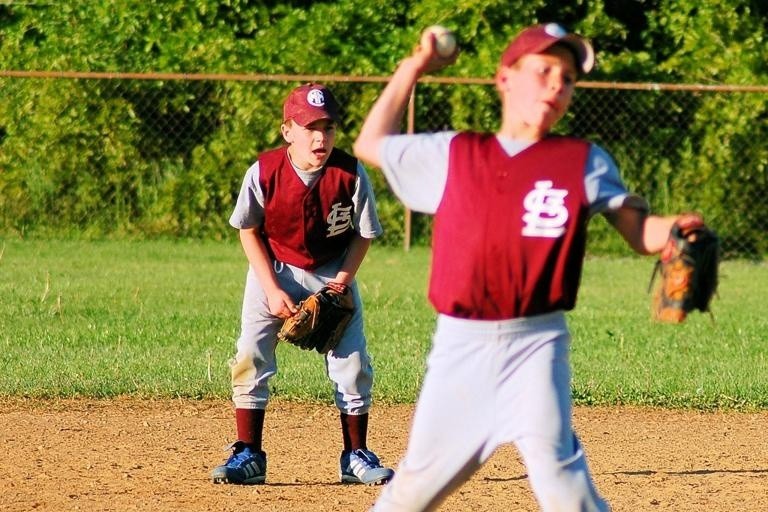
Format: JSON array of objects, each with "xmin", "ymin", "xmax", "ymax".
[{"xmin": 420, "ymin": 23, "xmax": 456, "ymax": 57}]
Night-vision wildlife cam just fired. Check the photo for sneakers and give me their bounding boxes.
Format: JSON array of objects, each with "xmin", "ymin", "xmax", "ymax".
[
  {"xmin": 212, "ymin": 439, "xmax": 268, "ymax": 485},
  {"xmin": 340, "ymin": 447, "xmax": 395, "ymax": 485}
]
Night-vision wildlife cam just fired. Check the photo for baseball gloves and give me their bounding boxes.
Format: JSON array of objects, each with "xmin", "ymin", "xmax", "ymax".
[
  {"xmin": 278, "ymin": 283, "xmax": 355, "ymax": 354},
  {"xmin": 648, "ymin": 213, "xmax": 728, "ymax": 324}
]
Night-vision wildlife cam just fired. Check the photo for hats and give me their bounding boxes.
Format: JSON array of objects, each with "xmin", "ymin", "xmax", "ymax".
[
  {"xmin": 499, "ymin": 22, "xmax": 596, "ymax": 80},
  {"xmin": 283, "ymin": 82, "xmax": 340, "ymax": 128}
]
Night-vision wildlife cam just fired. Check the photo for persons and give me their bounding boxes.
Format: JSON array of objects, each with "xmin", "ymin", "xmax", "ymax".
[
  {"xmin": 351, "ymin": 23, "xmax": 718, "ymax": 512},
  {"xmin": 210, "ymin": 85, "xmax": 395, "ymax": 484}
]
